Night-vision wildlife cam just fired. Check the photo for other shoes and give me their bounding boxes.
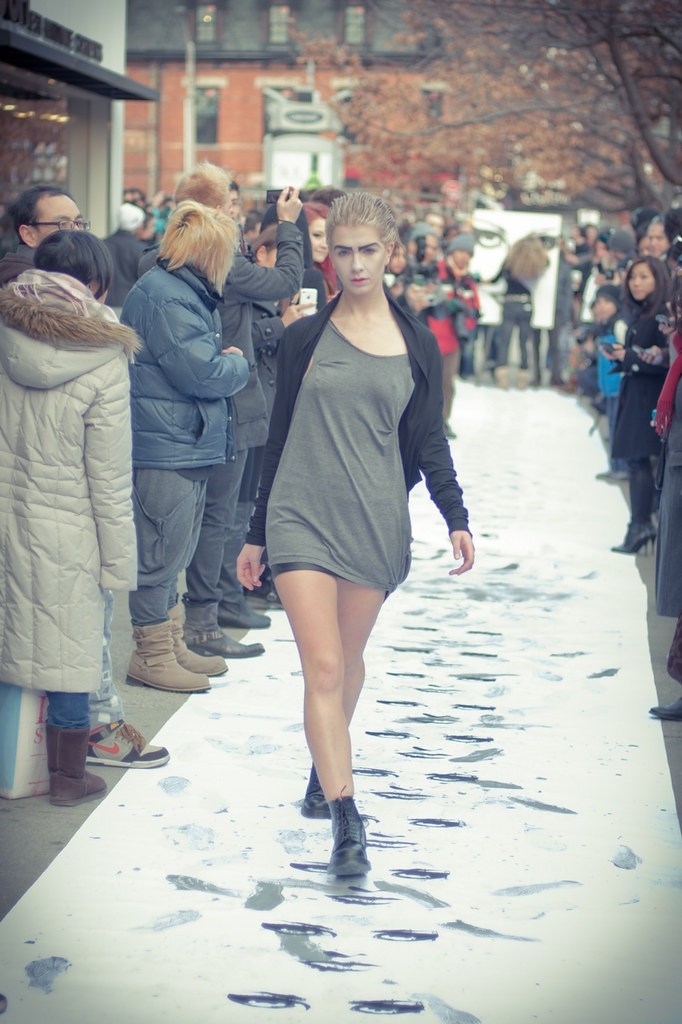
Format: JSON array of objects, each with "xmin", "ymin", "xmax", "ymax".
[
  {"xmin": 596, "ymin": 470, "xmax": 631, "ymax": 484},
  {"xmin": 443, "ymin": 423, "xmax": 455, "ymax": 439},
  {"xmin": 218, "ymin": 600, "xmax": 271, "ymax": 628},
  {"xmin": 243, "ymin": 581, "xmax": 282, "ymax": 610}
]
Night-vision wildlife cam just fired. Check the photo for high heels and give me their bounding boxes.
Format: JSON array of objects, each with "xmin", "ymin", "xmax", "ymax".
[{"xmin": 612, "ymin": 518, "xmax": 657, "ymax": 556}]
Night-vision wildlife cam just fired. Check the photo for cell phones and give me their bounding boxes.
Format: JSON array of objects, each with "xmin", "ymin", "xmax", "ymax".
[
  {"xmin": 631, "ymin": 344, "xmax": 656, "ymax": 362},
  {"xmin": 655, "ymin": 314, "xmax": 673, "ymax": 328},
  {"xmin": 299, "ymin": 289, "xmax": 317, "ymax": 316},
  {"xmin": 267, "ymin": 190, "xmax": 290, "ymax": 204}
]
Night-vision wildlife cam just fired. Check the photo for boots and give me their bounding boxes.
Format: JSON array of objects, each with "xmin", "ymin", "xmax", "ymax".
[
  {"xmin": 126, "ymin": 603, "xmax": 230, "ymax": 693},
  {"xmin": 180, "ymin": 594, "xmax": 266, "ymax": 657},
  {"xmin": 45, "ymin": 717, "xmax": 107, "ymax": 807}
]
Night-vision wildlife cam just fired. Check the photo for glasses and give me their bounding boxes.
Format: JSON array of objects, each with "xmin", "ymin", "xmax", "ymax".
[{"xmin": 29, "ymin": 219, "xmax": 91, "ymax": 232}]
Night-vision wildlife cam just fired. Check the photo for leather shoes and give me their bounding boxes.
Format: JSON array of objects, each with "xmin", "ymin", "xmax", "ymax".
[
  {"xmin": 302, "ymin": 762, "xmax": 332, "ymax": 820},
  {"xmin": 327, "ymin": 796, "xmax": 371, "ymax": 876},
  {"xmin": 648, "ymin": 697, "xmax": 682, "ymax": 721}
]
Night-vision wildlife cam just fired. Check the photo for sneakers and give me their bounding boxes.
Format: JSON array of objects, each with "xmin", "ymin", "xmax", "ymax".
[{"xmin": 84, "ymin": 717, "xmax": 169, "ymax": 767}]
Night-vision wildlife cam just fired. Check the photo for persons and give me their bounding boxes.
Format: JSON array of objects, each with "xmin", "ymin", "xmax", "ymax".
[
  {"xmin": 102, "ymin": 162, "xmax": 682, "ymax": 724},
  {"xmin": 0, "ymin": 227, "xmax": 140, "ymax": 807},
  {"xmin": 0, "ymin": 183, "xmax": 172, "ymax": 770},
  {"xmin": 236, "ymin": 190, "xmax": 476, "ymax": 879}
]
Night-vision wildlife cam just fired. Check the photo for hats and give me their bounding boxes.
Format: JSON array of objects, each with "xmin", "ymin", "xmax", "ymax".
[
  {"xmin": 118, "ymin": 202, "xmax": 146, "ymax": 231},
  {"xmin": 446, "ymin": 231, "xmax": 474, "ymax": 259},
  {"xmin": 405, "ymin": 220, "xmax": 441, "ymax": 244},
  {"xmin": 628, "ymin": 206, "xmax": 658, "ymax": 237},
  {"xmin": 259, "ymin": 204, "xmax": 314, "ymax": 269}
]
